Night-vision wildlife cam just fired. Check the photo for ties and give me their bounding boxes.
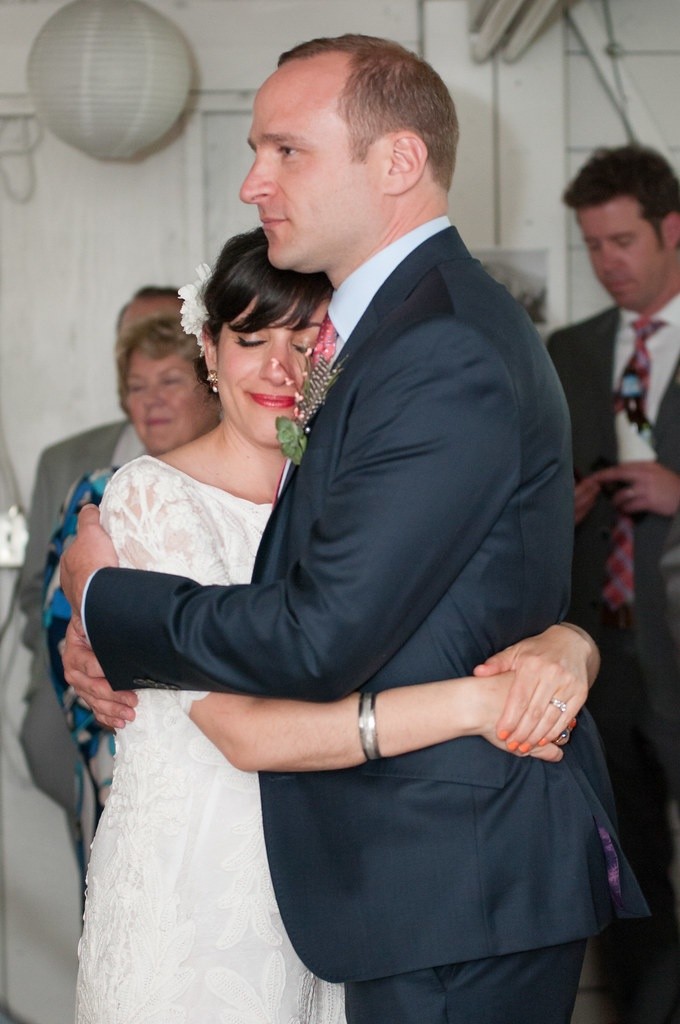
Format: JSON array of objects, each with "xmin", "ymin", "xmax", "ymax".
[
  {"xmin": 272, "ymin": 311, "xmax": 338, "ymax": 505},
  {"xmin": 602, "ymin": 318, "xmax": 666, "ymax": 608}
]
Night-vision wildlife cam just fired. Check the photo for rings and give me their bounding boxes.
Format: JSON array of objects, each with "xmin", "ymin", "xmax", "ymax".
[
  {"xmin": 555, "ymin": 729, "xmax": 568, "ymax": 742},
  {"xmin": 550, "ymin": 699, "xmax": 566, "ymax": 712}
]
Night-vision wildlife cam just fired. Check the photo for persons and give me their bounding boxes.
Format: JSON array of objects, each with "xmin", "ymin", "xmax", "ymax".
[
  {"xmin": 37, "ymin": 314, "xmax": 223, "ymax": 944},
  {"xmin": 10, "ymin": 283, "xmax": 186, "ymax": 866},
  {"xmin": 540, "ymin": 145, "xmax": 680, "ymax": 1024},
  {"xmin": 63, "ymin": 36, "xmax": 654, "ymax": 1024},
  {"xmin": 77, "ymin": 226, "xmax": 600, "ymax": 1024}
]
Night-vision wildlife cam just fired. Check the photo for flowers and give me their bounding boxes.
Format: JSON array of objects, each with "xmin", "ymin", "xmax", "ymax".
[
  {"xmin": 270, "ymin": 348, "xmax": 350, "ymax": 467},
  {"xmin": 176, "ymin": 264, "xmax": 215, "ymax": 359}
]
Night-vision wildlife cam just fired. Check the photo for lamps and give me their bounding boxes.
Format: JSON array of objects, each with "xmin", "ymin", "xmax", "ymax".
[{"xmin": 469, "ymin": 0, "xmax": 557, "ymax": 62}]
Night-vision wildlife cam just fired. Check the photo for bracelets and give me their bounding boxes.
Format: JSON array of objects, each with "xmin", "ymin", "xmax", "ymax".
[{"xmin": 357, "ymin": 691, "xmax": 381, "ymax": 759}]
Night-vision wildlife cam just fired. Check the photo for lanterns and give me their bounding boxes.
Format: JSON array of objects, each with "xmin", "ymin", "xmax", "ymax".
[{"xmin": 24, "ymin": 0, "xmax": 191, "ymax": 161}]
